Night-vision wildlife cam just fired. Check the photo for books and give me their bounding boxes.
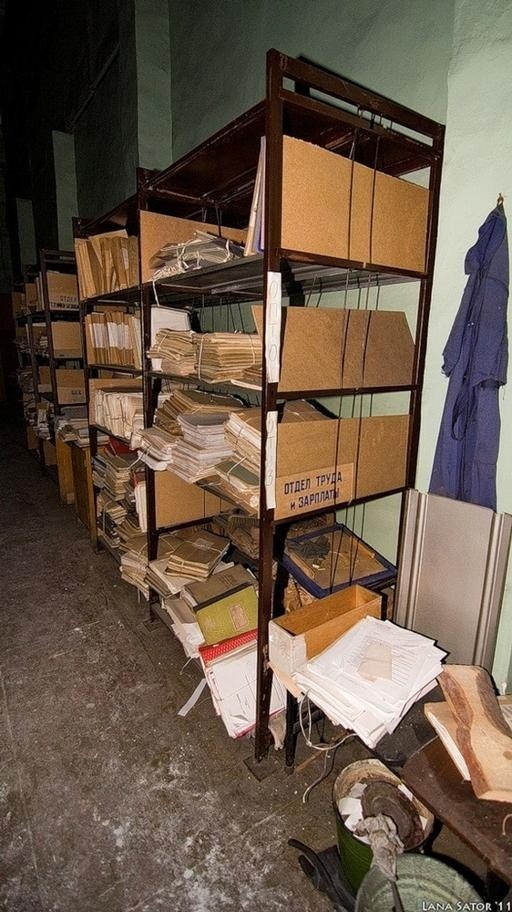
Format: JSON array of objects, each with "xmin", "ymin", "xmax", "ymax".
[
  {"xmin": 12, "ymin": 134, "xmax": 451, "ymax": 744},
  {"xmin": 424, "ymin": 695, "xmax": 512, "ymax": 782}
]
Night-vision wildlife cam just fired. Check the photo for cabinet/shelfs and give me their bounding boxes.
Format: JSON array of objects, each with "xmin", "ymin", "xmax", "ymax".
[
  {"xmin": 72, "ymin": 44, "xmax": 447, "ymax": 774},
  {"xmin": 6, "ymin": 257, "xmax": 84, "ymax": 515}
]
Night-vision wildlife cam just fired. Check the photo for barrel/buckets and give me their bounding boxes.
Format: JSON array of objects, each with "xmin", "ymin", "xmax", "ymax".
[
  {"xmin": 332, "ymin": 756, "xmax": 437, "ymax": 892},
  {"xmin": 353, "ymin": 852, "xmax": 487, "ymax": 912}
]
